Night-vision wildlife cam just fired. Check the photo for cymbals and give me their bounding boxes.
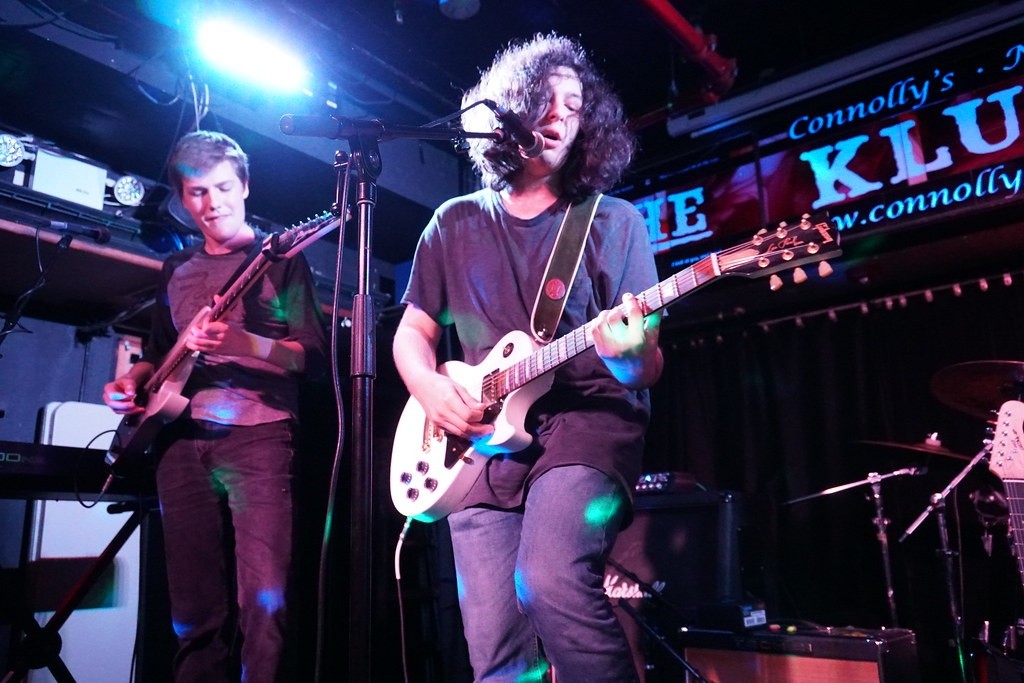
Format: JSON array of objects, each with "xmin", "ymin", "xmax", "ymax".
[
  {"xmin": 854, "ymin": 436, "xmax": 983, "ymax": 463},
  {"xmin": 785, "ymin": 465, "xmax": 909, "ymax": 506},
  {"xmin": 930, "ymin": 358, "xmax": 1024, "ymax": 416}
]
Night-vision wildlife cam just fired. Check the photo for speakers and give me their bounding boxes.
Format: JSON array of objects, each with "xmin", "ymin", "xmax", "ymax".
[
  {"xmin": 603, "ymin": 487, "xmax": 745, "ymax": 683},
  {"xmin": 157, "ymin": 186, "xmax": 201, "ymax": 235},
  {"xmin": 673, "ymin": 620, "xmax": 920, "ymax": 683}
]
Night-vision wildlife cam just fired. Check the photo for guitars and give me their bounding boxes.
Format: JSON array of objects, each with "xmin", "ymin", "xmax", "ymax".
[
  {"xmin": 982, "ymin": 399, "xmax": 1024, "ymax": 587},
  {"xmin": 99, "ymin": 197, "xmax": 352, "ymax": 488},
  {"xmin": 387, "ymin": 207, "xmax": 842, "ymax": 524}
]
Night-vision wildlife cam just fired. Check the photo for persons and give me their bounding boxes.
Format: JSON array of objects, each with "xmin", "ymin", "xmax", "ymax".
[
  {"xmin": 392, "ymin": 33, "xmax": 666, "ymax": 683},
  {"xmin": 103, "ymin": 131, "xmax": 325, "ymax": 683}
]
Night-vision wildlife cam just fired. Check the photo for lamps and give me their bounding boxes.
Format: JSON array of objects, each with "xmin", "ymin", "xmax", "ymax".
[{"xmin": 0, "ymin": 134, "xmax": 145, "ymax": 213}]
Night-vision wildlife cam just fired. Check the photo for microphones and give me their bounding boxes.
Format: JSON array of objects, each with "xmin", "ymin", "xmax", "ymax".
[
  {"xmin": 42, "ymin": 220, "xmax": 111, "ymax": 244},
  {"xmin": 484, "ymin": 99, "xmax": 545, "ymax": 159}
]
